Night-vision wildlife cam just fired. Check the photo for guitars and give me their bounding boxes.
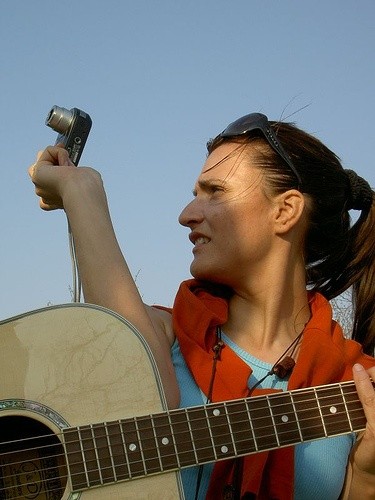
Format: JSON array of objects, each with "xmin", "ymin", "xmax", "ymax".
[{"xmin": 1, "ymin": 303, "xmax": 374, "ymax": 500}]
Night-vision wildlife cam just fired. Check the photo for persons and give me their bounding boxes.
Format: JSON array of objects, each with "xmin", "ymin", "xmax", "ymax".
[{"xmin": 28, "ymin": 111, "xmax": 375, "ymax": 500}]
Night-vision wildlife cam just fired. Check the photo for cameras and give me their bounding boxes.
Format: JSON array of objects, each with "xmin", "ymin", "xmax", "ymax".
[{"xmin": 44, "ymin": 105, "xmax": 93, "ymax": 167}]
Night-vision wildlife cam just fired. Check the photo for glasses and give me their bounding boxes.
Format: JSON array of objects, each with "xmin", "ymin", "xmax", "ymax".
[{"xmin": 210, "ymin": 112, "xmax": 307, "ymax": 192}]
